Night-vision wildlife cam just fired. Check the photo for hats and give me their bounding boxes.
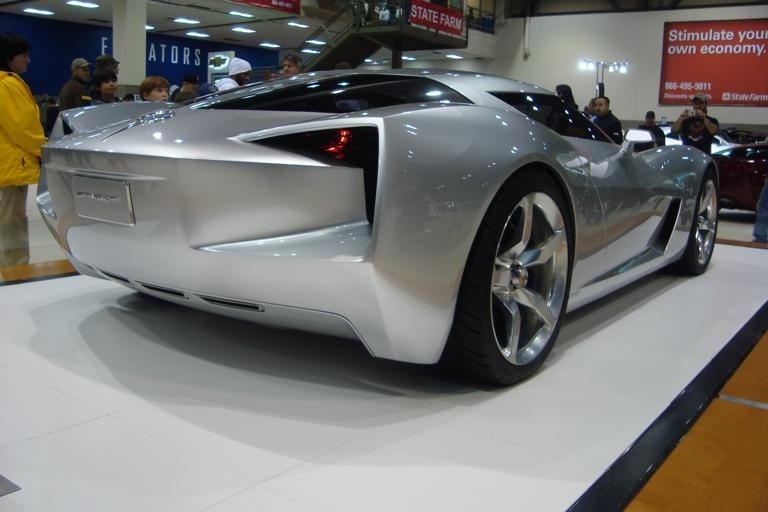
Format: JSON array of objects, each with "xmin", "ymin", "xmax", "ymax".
[
  {"xmin": 199, "ymin": 82, "xmax": 219, "ymax": 96},
  {"xmin": 228, "ymin": 57, "xmax": 252, "ymax": 76},
  {"xmin": 71, "ymin": 57, "xmax": 92, "ymax": 70},
  {"xmin": 692, "ymin": 93, "xmax": 708, "ymax": 104},
  {"xmin": 183, "ymin": 66, "xmax": 201, "ymax": 84}
]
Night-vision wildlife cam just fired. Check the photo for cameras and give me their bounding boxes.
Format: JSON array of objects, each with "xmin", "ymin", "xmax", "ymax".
[{"xmin": 688, "ymin": 109, "xmax": 697, "ymax": 117}]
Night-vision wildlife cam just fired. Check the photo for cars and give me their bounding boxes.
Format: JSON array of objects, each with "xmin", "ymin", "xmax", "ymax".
[{"xmin": 653, "ymin": 115, "xmax": 768, "ymax": 209}]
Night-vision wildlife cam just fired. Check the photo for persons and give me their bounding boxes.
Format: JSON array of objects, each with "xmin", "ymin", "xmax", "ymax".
[
  {"xmin": 90, "ymin": 65, "xmax": 121, "ymax": 107},
  {"xmin": 36, "ymin": 92, "xmax": 49, "ymax": 107},
  {"xmin": 349, "ymin": 0, "xmax": 444, "ymax": 34},
  {"xmin": 92, "ymin": 53, "xmax": 120, "ymax": 75},
  {"xmin": 167, "ymin": 72, "xmax": 218, "ymax": 103},
  {"xmin": 58, "ymin": 57, "xmax": 91, "ymax": 135},
  {"xmin": 138, "ymin": 74, "xmax": 170, "ymax": 103},
  {"xmin": 669, "ymin": 91, "xmax": 719, "ymax": 157},
  {"xmin": 213, "ymin": 57, "xmax": 252, "ymax": 91},
  {"xmin": 588, "ymin": 95, "xmax": 622, "ymax": 145},
  {"xmin": 544, "ymin": 83, "xmax": 577, "ymax": 136},
  {"xmin": 632, "ymin": 110, "xmax": 665, "ymax": 153},
  {"xmin": 0, "ymin": 31, "xmax": 47, "ymax": 268},
  {"xmin": 740, "ymin": 133, "xmax": 767, "ymax": 243},
  {"xmin": 45, "ymin": 96, "xmax": 60, "ymax": 137},
  {"xmin": 587, "ymin": 97, "xmax": 597, "ymax": 124},
  {"xmin": 270, "ymin": 52, "xmax": 303, "ymax": 78}
]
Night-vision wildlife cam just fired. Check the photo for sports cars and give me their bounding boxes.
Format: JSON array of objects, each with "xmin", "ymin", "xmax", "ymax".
[{"xmin": 34, "ymin": 59, "xmax": 723, "ymax": 391}]
